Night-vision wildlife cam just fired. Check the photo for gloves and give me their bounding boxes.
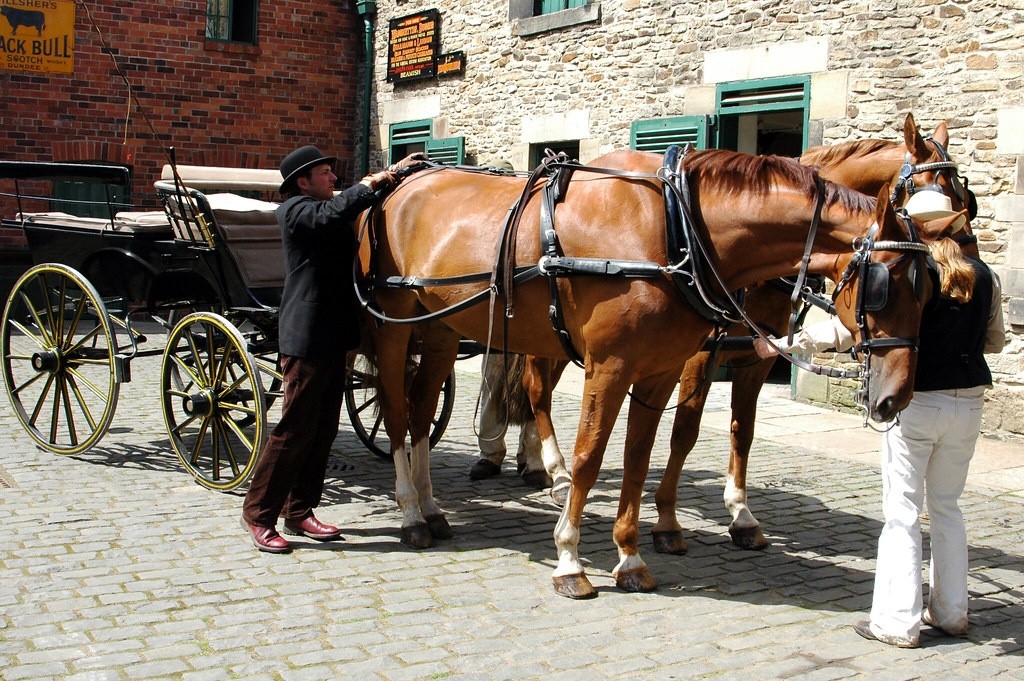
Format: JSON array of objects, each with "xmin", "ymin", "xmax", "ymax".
[
  {"xmin": 389, "ymin": 152, "xmax": 429, "ymax": 174},
  {"xmin": 362, "ymin": 170, "xmax": 402, "ymax": 190}
]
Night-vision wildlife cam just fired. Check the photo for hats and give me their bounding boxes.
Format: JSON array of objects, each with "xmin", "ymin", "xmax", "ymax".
[
  {"xmin": 279, "ymin": 145, "xmax": 337, "ymax": 193},
  {"xmin": 904, "ymin": 190, "xmax": 966, "ymax": 234}
]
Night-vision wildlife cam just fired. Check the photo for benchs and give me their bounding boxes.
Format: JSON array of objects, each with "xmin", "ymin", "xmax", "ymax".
[
  {"xmin": 155, "ymin": 179, "xmax": 343, "ymax": 245},
  {"xmin": 0, "ymin": 158, "xmax": 171, "ymax": 234}
]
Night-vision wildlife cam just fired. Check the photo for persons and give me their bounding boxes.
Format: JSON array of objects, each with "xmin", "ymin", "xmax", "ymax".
[
  {"xmin": 857, "ymin": 188, "xmax": 1004, "ymax": 648},
  {"xmin": 241, "ymin": 145, "xmax": 430, "ymax": 552}
]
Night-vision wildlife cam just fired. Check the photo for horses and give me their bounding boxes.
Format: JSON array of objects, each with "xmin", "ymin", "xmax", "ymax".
[{"xmin": 347, "ymin": 110, "xmax": 983, "ymax": 602}]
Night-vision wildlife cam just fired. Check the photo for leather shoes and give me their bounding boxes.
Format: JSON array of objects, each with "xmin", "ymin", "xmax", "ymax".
[
  {"xmin": 853, "ymin": 621, "xmax": 877, "ymax": 639},
  {"xmin": 283, "ymin": 510, "xmax": 341, "ymax": 539},
  {"xmin": 920, "ymin": 606, "xmax": 969, "ymax": 637},
  {"xmin": 239, "ymin": 516, "xmax": 289, "ymax": 552}
]
